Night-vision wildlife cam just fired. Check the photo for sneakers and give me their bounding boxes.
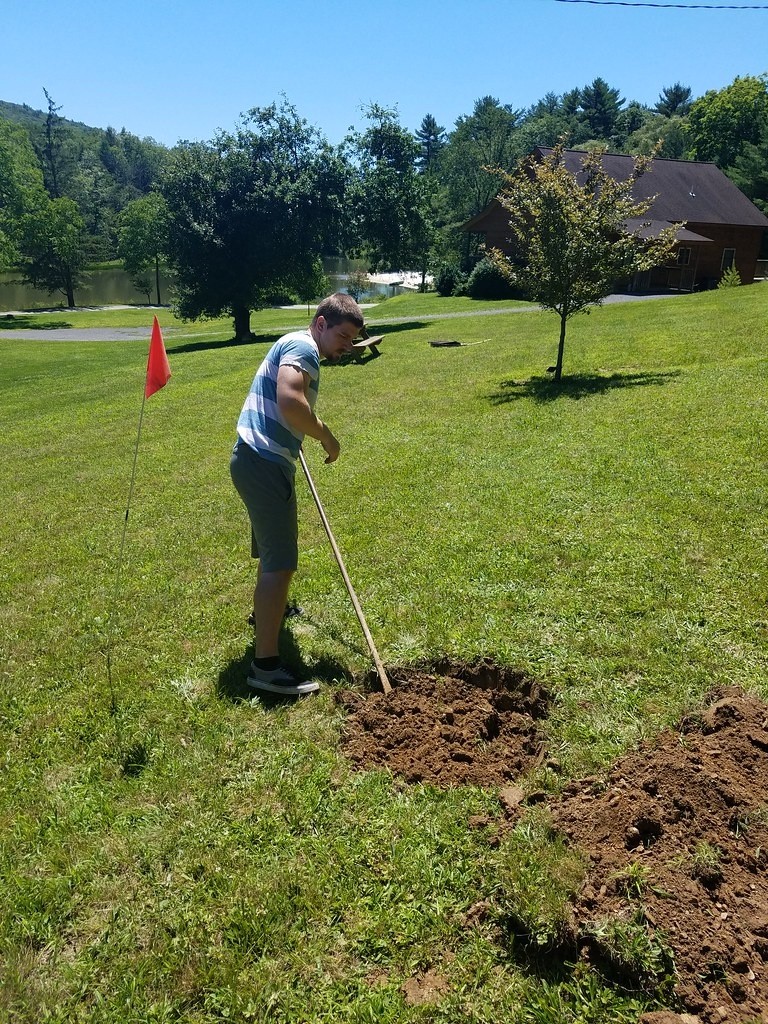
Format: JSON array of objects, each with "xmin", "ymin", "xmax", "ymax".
[
  {"xmin": 247, "ymin": 660, "xmax": 319, "ymax": 694},
  {"xmin": 248, "ymin": 604, "xmax": 304, "ymax": 623}
]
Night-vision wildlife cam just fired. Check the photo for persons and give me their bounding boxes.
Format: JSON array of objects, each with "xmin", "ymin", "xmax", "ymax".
[{"xmin": 229, "ymin": 292, "xmax": 364, "ymax": 695}]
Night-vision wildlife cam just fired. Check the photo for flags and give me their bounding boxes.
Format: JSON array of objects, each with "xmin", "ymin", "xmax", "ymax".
[{"xmin": 145, "ymin": 315, "xmax": 172, "ymax": 399}]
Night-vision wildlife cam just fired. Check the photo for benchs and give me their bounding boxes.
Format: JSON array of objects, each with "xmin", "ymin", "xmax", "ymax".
[{"xmin": 352, "ymin": 334, "xmax": 386, "ymax": 348}]
